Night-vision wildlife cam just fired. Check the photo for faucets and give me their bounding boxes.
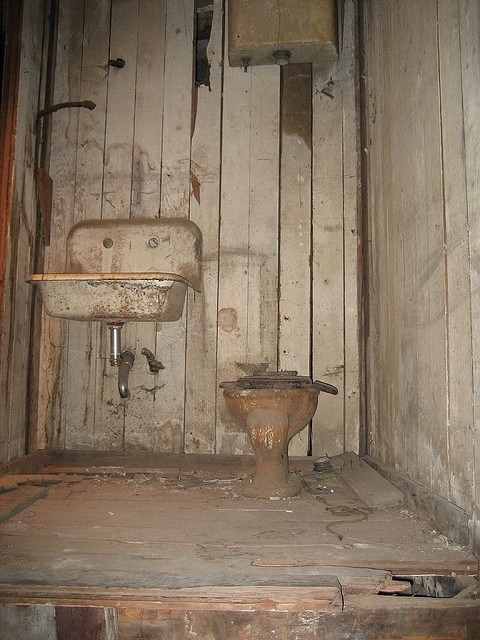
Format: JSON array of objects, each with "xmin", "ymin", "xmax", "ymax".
[{"xmin": 37, "ymin": 99, "xmax": 96, "ymax": 112}]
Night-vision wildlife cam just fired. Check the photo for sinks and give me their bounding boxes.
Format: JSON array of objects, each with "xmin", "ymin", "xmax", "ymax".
[{"xmin": 25, "ymin": 218, "xmax": 203, "ymax": 322}]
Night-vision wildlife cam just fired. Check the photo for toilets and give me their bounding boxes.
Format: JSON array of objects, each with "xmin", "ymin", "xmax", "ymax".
[{"xmin": 218, "ymin": 0, "xmax": 339, "ymax": 501}]
